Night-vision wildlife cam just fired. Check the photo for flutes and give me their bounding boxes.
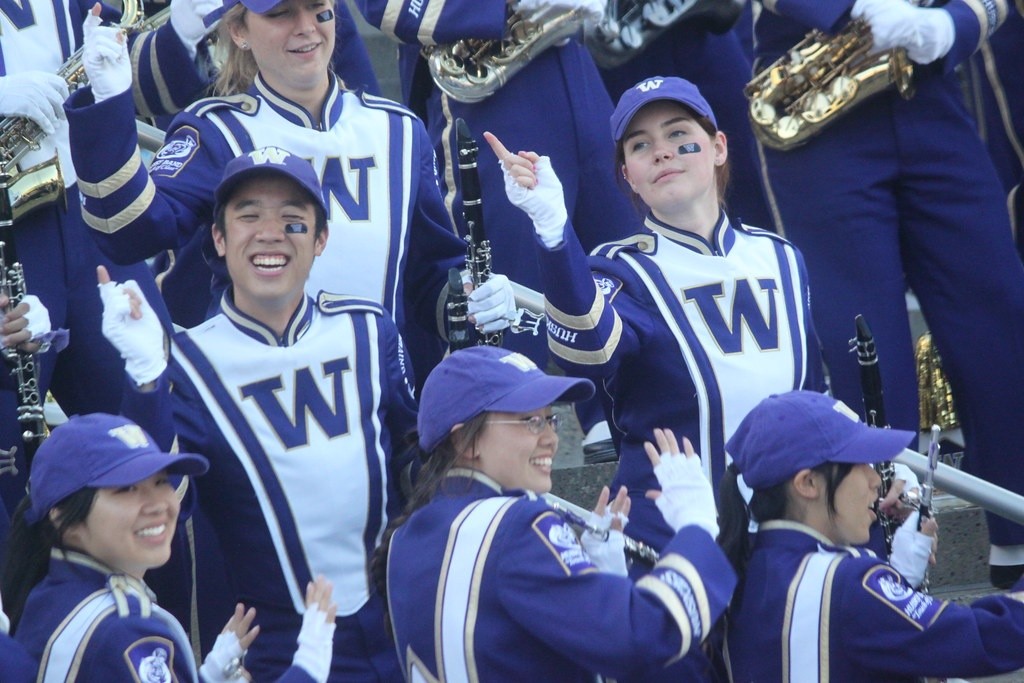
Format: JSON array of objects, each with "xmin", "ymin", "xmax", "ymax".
[
  {"xmin": 540, "ymin": 492, "xmax": 661, "ymax": 562},
  {"xmin": 914, "ymin": 422, "xmax": 942, "ymax": 594},
  {"xmin": 0, "ymin": 168, "xmax": 51, "ymax": 473},
  {"xmin": 847, "ymin": 310, "xmax": 903, "ymax": 562},
  {"xmin": 443, "ymin": 266, "xmax": 470, "ymax": 352},
  {"xmin": 452, "ymin": 113, "xmax": 505, "ymax": 349}
]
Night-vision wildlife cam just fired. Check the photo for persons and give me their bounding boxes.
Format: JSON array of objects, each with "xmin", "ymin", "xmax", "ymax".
[
  {"xmin": 355, "ymin": 1, "xmax": 1024, "ymax": 590},
  {"xmin": 718, "ymin": 390, "xmax": 1024, "ymax": 683},
  {"xmin": 97, "ymin": 146, "xmax": 426, "ymax": 683},
  {"xmin": 62, "ymin": 0, "xmax": 516, "ymax": 346},
  {"xmin": 0, "ymin": 412, "xmax": 337, "ymax": 683},
  {"xmin": 484, "ymin": 76, "xmax": 922, "ymax": 683},
  {"xmin": 1, "ymin": 0, "xmax": 379, "ymax": 633},
  {"xmin": 372, "ymin": 347, "xmax": 737, "ymax": 683}
]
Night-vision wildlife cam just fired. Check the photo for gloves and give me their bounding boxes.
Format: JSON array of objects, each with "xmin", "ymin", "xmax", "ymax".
[
  {"xmin": 516, "ymin": 1, "xmax": 607, "ymax": 48},
  {"xmin": 170, "ymin": 0, "xmax": 225, "ymax": 64},
  {"xmin": 852, "ymin": 0, "xmax": 956, "ymax": 66}
]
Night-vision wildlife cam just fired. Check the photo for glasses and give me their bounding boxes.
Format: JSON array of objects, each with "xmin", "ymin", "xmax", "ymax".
[{"xmin": 468, "ymin": 414, "xmax": 559, "ymax": 433}]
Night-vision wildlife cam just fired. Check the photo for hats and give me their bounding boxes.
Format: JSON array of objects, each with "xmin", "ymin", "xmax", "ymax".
[
  {"xmin": 609, "ymin": 74, "xmax": 718, "ymax": 153},
  {"xmin": 417, "ymin": 344, "xmax": 596, "ymax": 453},
  {"xmin": 23, "ymin": 412, "xmax": 210, "ymax": 528},
  {"xmin": 224, "ymin": 0, "xmax": 283, "ymax": 14},
  {"xmin": 723, "ymin": 391, "xmax": 916, "ymax": 489},
  {"xmin": 215, "ymin": 147, "xmax": 328, "ymax": 219}
]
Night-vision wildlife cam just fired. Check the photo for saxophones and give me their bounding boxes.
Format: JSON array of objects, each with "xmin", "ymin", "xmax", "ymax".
[
  {"xmin": 585, "ymin": 0, "xmax": 695, "ymax": 69},
  {"xmin": 0, "ymin": 0, "xmax": 174, "ymax": 178},
  {"xmin": 741, "ymin": 13, "xmax": 918, "ymax": 152},
  {"xmin": 420, "ymin": 0, "xmax": 590, "ymax": 105}
]
